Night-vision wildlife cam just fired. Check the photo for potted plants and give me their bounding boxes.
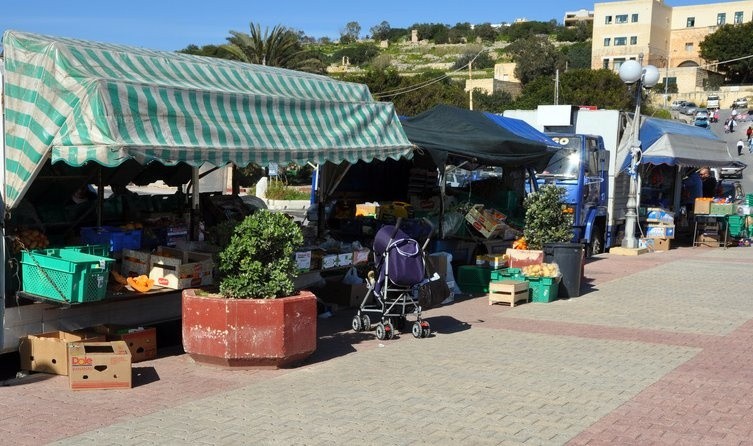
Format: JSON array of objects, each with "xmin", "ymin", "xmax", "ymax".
[
  {"xmin": 507, "ymin": 184, "xmax": 574, "ymax": 267},
  {"xmin": 182, "ymin": 209, "xmax": 317, "ymax": 369}
]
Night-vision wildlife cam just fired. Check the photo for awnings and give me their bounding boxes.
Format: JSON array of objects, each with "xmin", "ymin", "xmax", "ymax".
[{"xmin": 613, "ymin": 113, "xmax": 734, "ymax": 180}]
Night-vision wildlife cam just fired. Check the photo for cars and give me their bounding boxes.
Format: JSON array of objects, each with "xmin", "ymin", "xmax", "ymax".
[
  {"xmin": 678, "ymin": 102, "xmax": 698, "ymax": 116},
  {"xmin": 692, "ymin": 109, "xmax": 709, "ymax": 120},
  {"xmin": 736, "ymin": 97, "xmax": 748, "ymax": 109},
  {"xmin": 720, "ymin": 166, "xmax": 742, "ymax": 177},
  {"xmin": 695, "ymin": 117, "xmax": 708, "ymax": 129},
  {"xmin": 670, "ymin": 99, "xmax": 686, "ymax": 111}
]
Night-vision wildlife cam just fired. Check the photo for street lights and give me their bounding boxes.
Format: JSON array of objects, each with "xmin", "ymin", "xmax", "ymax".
[{"xmin": 608, "ymin": 53, "xmax": 661, "ymax": 257}]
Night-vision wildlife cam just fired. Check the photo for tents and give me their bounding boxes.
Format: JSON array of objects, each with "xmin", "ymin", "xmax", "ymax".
[
  {"xmin": 0, "ymin": 32, "xmax": 418, "ymax": 354},
  {"xmin": 318, "ymin": 105, "xmax": 565, "ymax": 244}
]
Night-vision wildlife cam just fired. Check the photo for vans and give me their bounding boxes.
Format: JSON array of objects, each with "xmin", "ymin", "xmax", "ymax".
[{"xmin": 438, "ymin": 173, "xmax": 465, "ymax": 189}]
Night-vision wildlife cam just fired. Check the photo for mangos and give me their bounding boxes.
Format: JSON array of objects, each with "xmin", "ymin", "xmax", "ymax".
[{"xmin": 11, "ymin": 228, "xmax": 49, "ymax": 254}]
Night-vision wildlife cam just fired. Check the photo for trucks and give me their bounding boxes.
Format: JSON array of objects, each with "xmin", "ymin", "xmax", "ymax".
[
  {"xmin": 706, "ymin": 95, "xmax": 721, "ymax": 110},
  {"xmin": 469, "ymin": 124, "xmax": 675, "ymax": 259}
]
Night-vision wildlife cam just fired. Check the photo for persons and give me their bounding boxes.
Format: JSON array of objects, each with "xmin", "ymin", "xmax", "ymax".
[
  {"xmin": 713, "ymin": 101, "xmax": 753, "ymax": 155},
  {"xmin": 71, "ymin": 184, "xmax": 138, "ymax": 205},
  {"xmin": 682, "ymin": 167, "xmax": 718, "ymax": 237}
]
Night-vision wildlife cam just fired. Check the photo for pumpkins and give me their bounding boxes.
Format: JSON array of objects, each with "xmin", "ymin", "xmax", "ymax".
[{"xmin": 125, "ymin": 221, "xmax": 143, "ymax": 230}]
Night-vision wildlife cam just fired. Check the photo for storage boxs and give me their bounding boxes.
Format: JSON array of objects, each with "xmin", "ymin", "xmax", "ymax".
[
  {"xmin": 293, "ymin": 248, "xmax": 370, "ymax": 274},
  {"xmin": 407, "ymin": 167, "xmax": 456, "ymax": 211},
  {"xmin": 465, "ymin": 204, "xmax": 505, "ymax": 239},
  {"xmin": 418, "ymin": 256, "xmax": 450, "ymax": 306},
  {"xmin": 458, "ymin": 248, "xmax": 559, "ymax": 308},
  {"xmin": 694, "ymin": 193, "xmax": 753, "ymax": 248},
  {"xmin": 20, "ymin": 225, "xmax": 214, "ymax": 303},
  {"xmin": 19, "ymin": 324, "xmax": 158, "ymax": 389},
  {"xmin": 340, "ymin": 217, "xmax": 375, "ymax": 234},
  {"xmin": 322, "ymin": 276, "xmax": 373, "ymax": 307},
  {"xmin": 38, "ymin": 192, "xmax": 222, "ymax": 222},
  {"xmin": 639, "ymin": 207, "xmax": 675, "ymax": 250}
]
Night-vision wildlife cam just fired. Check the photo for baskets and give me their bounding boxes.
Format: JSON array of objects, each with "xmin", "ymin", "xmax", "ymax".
[{"xmin": 82, "ymin": 226, "xmax": 141, "ymax": 252}]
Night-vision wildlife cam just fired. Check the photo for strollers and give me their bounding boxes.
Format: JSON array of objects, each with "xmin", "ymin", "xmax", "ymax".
[{"xmin": 351, "ymin": 215, "xmax": 438, "ymax": 341}]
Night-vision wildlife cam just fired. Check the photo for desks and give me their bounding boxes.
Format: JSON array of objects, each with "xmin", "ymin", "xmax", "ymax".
[{"xmin": 693, "ymin": 214, "xmax": 729, "ymax": 248}]
